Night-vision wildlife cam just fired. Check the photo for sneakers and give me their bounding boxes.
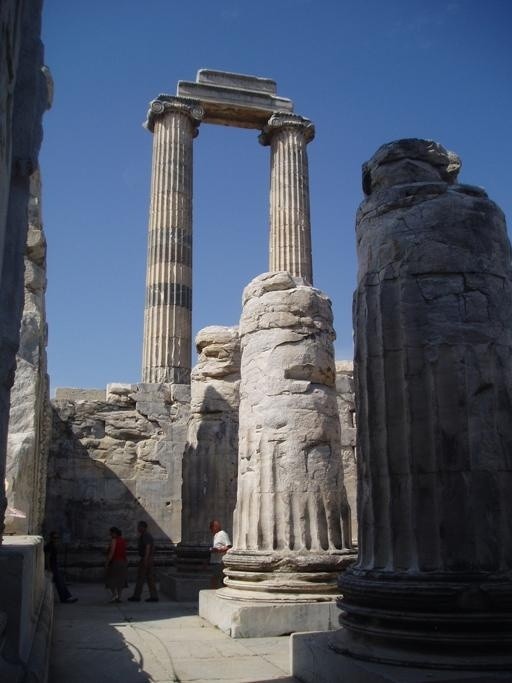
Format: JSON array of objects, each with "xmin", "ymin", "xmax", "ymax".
[
  {"xmin": 64, "ymin": 597, "xmax": 78, "ymax": 603},
  {"xmin": 128, "ymin": 596, "xmax": 159, "ymax": 602}
]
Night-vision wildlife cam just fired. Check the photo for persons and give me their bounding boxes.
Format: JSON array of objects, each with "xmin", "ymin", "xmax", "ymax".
[
  {"xmin": 210, "ymin": 520, "xmax": 232, "ymax": 589},
  {"xmin": 44, "ymin": 532, "xmax": 78, "ymax": 603},
  {"xmin": 129, "ymin": 521, "xmax": 159, "ymax": 602},
  {"xmin": 104, "ymin": 527, "xmax": 129, "ymax": 602}
]
